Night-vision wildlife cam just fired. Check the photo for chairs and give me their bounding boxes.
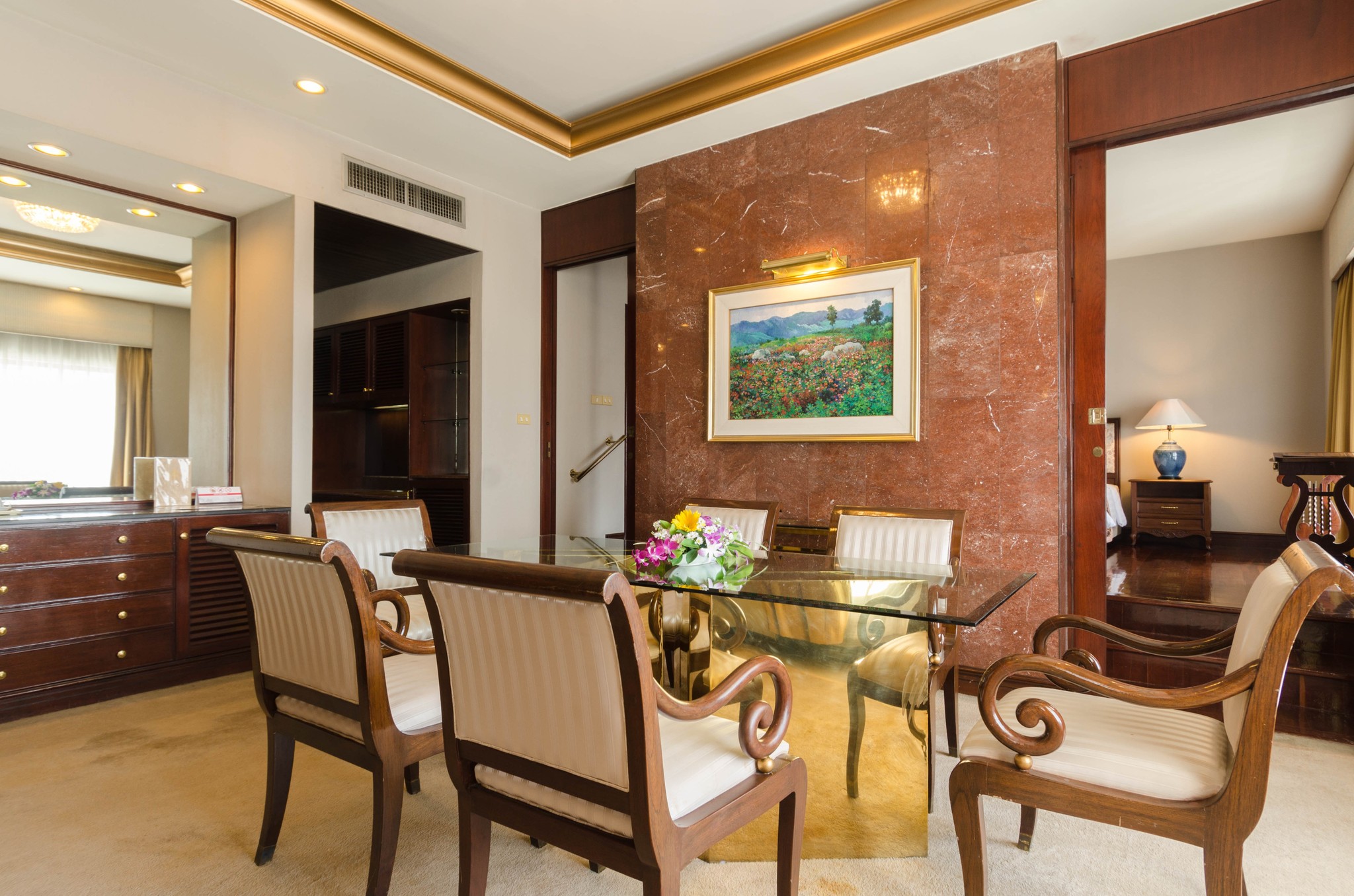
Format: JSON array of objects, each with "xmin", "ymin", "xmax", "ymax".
[
  {"xmin": 206, "ymin": 526, "xmax": 549, "ymax": 895},
  {"xmin": 391, "ymin": 547, "xmax": 808, "ymax": 896},
  {"xmin": 679, "ymin": 495, "xmax": 784, "ymax": 551},
  {"xmin": 824, "ymin": 505, "xmax": 969, "ymax": 758},
  {"xmin": 844, "ymin": 579, "xmax": 928, "ymax": 800},
  {"xmin": 948, "ymin": 539, "xmax": 1354, "ymax": 896},
  {"xmin": 304, "ymin": 499, "xmax": 433, "ymax": 661}
]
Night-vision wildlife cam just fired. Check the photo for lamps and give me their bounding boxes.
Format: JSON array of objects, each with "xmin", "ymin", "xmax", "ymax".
[
  {"xmin": 12, "ymin": 199, "xmax": 100, "ymax": 233},
  {"xmin": 1134, "ymin": 398, "xmax": 1208, "ymax": 480},
  {"xmin": 865, "ymin": 165, "xmax": 944, "ymax": 215},
  {"xmin": 760, "ymin": 246, "xmax": 847, "ymax": 280}
]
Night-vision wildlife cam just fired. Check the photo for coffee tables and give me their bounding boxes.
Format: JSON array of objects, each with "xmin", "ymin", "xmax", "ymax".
[{"xmin": 564, "ymin": 546, "xmax": 1038, "ymax": 865}]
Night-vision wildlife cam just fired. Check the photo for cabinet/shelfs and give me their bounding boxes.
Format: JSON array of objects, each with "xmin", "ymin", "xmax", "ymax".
[
  {"xmin": 407, "ymin": 297, "xmax": 471, "ymax": 478},
  {"xmin": 313, "ymin": 310, "xmax": 408, "ymax": 411},
  {"xmin": 412, "ymin": 477, "xmax": 470, "ymax": 547},
  {"xmin": 0, "ymin": 504, "xmax": 292, "ymax": 725}
]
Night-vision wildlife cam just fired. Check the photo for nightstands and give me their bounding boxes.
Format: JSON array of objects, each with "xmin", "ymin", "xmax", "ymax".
[{"xmin": 1128, "ymin": 478, "xmax": 1214, "ymax": 551}]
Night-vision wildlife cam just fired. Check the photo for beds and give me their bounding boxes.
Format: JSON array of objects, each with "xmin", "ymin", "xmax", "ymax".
[{"xmin": 1106, "ymin": 483, "xmax": 1127, "ymax": 544}]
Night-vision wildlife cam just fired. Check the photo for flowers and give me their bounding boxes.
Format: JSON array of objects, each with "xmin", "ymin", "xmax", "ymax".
[
  {"xmin": 631, "ymin": 507, "xmax": 769, "ymax": 570},
  {"xmin": 634, "ymin": 562, "xmax": 769, "ymax": 592}
]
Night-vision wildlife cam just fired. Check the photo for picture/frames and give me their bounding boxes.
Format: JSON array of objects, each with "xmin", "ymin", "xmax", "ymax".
[{"xmin": 706, "ymin": 256, "xmax": 921, "ymax": 442}]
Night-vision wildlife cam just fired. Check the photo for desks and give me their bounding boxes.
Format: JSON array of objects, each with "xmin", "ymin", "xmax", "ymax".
[{"xmin": 1269, "ymin": 452, "xmax": 1354, "ymax": 568}]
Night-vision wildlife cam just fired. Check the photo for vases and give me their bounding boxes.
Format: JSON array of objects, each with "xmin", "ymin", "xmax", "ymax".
[
  {"xmin": 663, "ymin": 561, "xmax": 726, "ymax": 583},
  {"xmin": 676, "ymin": 552, "xmax": 714, "ymax": 567}
]
